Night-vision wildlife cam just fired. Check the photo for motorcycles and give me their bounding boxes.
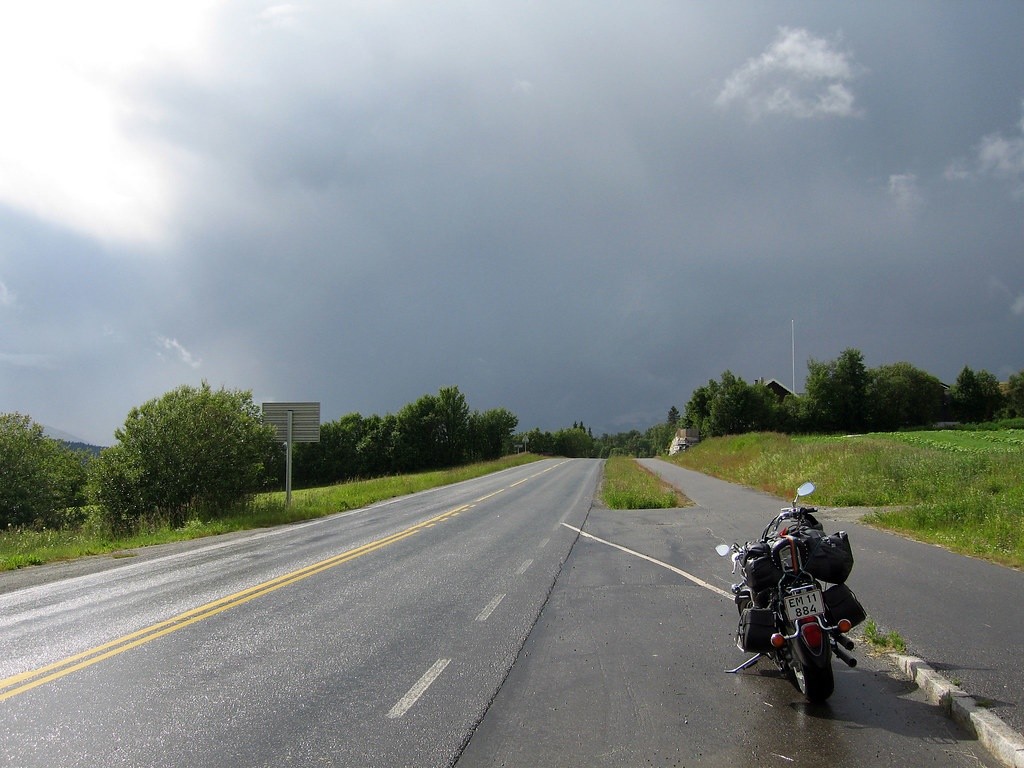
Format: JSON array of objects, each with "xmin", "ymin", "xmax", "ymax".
[{"xmin": 714, "ymin": 481, "xmax": 869, "ymax": 704}]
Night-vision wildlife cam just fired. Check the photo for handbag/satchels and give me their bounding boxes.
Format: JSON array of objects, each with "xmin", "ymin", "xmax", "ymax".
[
  {"xmin": 787, "ymin": 523, "xmax": 853, "ymax": 584},
  {"xmin": 740, "ymin": 542, "xmax": 781, "ymax": 592}
]
